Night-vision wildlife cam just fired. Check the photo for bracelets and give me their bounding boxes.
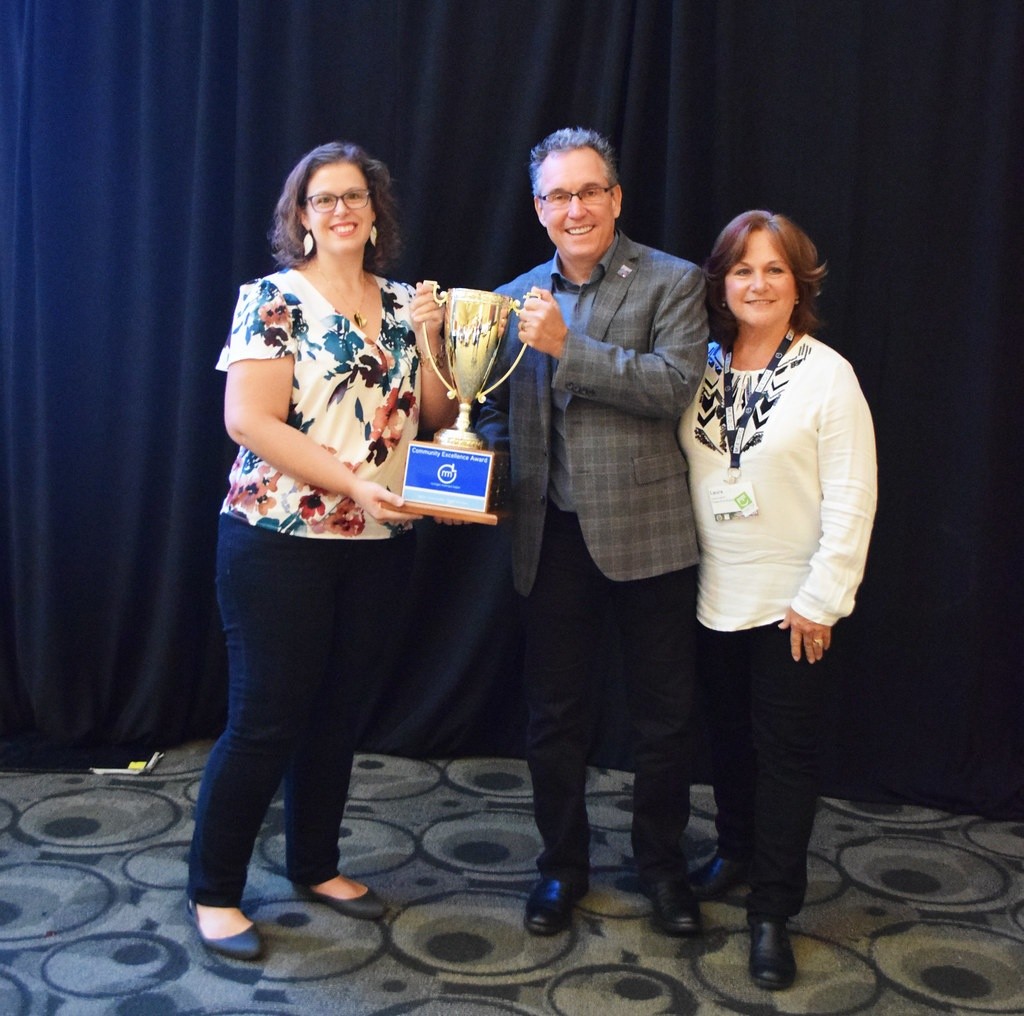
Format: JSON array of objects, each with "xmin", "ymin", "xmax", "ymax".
[{"xmin": 416, "ymin": 345, "xmax": 446, "ymax": 371}]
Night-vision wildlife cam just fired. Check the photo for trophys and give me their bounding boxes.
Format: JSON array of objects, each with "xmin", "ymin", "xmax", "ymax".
[{"xmin": 381, "ymin": 280, "xmax": 541, "ymax": 526}]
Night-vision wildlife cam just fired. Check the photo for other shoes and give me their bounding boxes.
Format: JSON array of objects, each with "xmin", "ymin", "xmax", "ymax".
[
  {"xmin": 292, "ymin": 874, "xmax": 387, "ymax": 920},
  {"xmin": 188, "ymin": 899, "xmax": 263, "ymax": 961}
]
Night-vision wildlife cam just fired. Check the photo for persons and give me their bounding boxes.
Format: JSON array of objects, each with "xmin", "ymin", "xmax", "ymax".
[
  {"xmin": 434, "ymin": 128, "xmax": 710, "ymax": 933},
  {"xmin": 183, "ymin": 140, "xmax": 445, "ymax": 961},
  {"xmin": 676, "ymin": 209, "xmax": 878, "ymax": 990}
]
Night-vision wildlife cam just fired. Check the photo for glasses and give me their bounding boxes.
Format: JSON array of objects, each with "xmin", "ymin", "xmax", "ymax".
[
  {"xmin": 537, "ymin": 181, "xmax": 620, "ymax": 205},
  {"xmin": 302, "ymin": 188, "xmax": 372, "ymax": 212}
]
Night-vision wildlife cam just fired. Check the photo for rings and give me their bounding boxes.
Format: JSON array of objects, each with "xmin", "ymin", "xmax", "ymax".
[{"xmin": 813, "ymin": 639, "xmax": 822, "ymax": 647}]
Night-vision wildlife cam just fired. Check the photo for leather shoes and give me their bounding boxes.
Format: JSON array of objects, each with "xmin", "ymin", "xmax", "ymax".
[
  {"xmin": 524, "ymin": 876, "xmax": 589, "ymax": 936},
  {"xmin": 639, "ymin": 865, "xmax": 702, "ymax": 935},
  {"xmin": 687, "ymin": 852, "xmax": 758, "ymax": 899},
  {"xmin": 745, "ymin": 913, "xmax": 797, "ymax": 991}
]
